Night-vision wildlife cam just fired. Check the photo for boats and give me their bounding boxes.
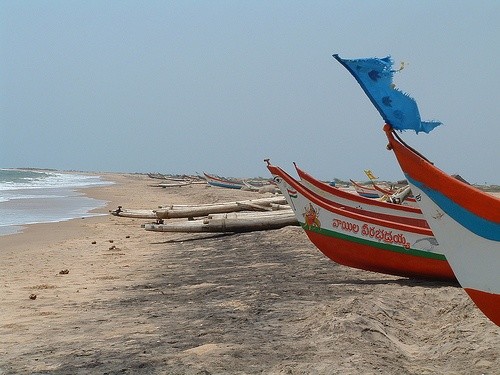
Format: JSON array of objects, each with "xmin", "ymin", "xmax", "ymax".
[
  {"xmin": 373, "ymin": 185, "xmax": 420, "ymax": 208},
  {"xmin": 194, "ymin": 170, "xmax": 271, "ymax": 189},
  {"xmin": 349, "ymin": 179, "xmax": 379, "ymax": 198},
  {"xmin": 264, "ymin": 157, "xmax": 457, "ymax": 283},
  {"xmin": 382, "ymin": 125, "xmax": 500, "ymax": 329},
  {"xmin": 292, "ymin": 161, "xmax": 430, "ymax": 231}
]
